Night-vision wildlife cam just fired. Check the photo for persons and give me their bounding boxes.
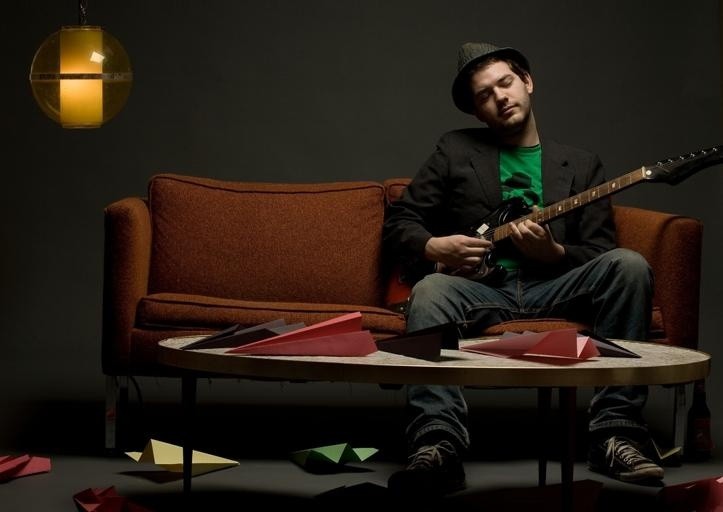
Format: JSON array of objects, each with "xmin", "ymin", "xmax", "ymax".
[{"xmin": 382, "ymin": 39, "xmax": 667, "ymax": 494}]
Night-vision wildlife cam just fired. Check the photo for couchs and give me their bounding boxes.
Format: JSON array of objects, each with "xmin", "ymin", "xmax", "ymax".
[{"xmin": 97, "ymin": 173, "xmax": 703, "ymax": 470}]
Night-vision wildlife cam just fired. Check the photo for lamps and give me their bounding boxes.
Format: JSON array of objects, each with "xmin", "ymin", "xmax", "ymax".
[{"xmin": 26, "ymin": 1, "xmax": 133, "ymax": 133}]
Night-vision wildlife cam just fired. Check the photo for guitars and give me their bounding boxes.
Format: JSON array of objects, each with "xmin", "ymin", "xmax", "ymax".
[{"xmin": 384, "ymin": 146, "xmax": 723, "ymax": 316}]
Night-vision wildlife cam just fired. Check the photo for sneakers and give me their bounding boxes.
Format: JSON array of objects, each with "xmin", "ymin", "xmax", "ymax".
[
  {"xmin": 387, "ymin": 442, "xmax": 466, "ymax": 501},
  {"xmin": 587, "ymin": 435, "xmax": 665, "ymax": 484}
]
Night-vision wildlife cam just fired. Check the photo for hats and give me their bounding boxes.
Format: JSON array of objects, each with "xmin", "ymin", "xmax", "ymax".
[{"xmin": 451, "ymin": 42, "xmax": 531, "ymax": 117}]
